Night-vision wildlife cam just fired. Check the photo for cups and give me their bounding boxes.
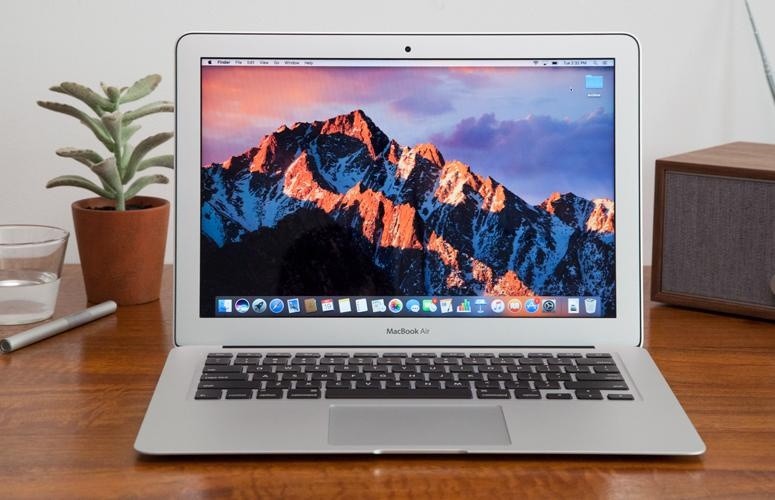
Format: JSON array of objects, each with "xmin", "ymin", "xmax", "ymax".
[{"xmin": 0, "ymin": 224, "xmax": 70, "ymax": 326}]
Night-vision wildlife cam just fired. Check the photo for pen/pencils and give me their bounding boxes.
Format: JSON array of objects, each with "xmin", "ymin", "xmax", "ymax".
[{"xmin": 0, "ymin": 300, "xmax": 118, "ymax": 353}]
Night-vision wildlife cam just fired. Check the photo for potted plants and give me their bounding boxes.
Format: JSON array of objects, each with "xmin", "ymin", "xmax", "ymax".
[{"xmin": 35, "ymin": 73, "xmax": 176, "ymax": 307}]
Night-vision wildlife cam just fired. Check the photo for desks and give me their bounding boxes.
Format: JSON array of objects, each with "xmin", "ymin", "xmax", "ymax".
[{"xmin": 0, "ymin": 259, "xmax": 775, "ymax": 500}]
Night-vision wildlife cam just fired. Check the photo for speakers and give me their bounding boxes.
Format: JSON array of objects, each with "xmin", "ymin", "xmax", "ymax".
[{"xmin": 651, "ymin": 137, "xmax": 775, "ymax": 322}]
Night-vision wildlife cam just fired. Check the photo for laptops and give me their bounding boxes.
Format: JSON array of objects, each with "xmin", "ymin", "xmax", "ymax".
[{"xmin": 131, "ymin": 31, "xmax": 708, "ymax": 455}]
